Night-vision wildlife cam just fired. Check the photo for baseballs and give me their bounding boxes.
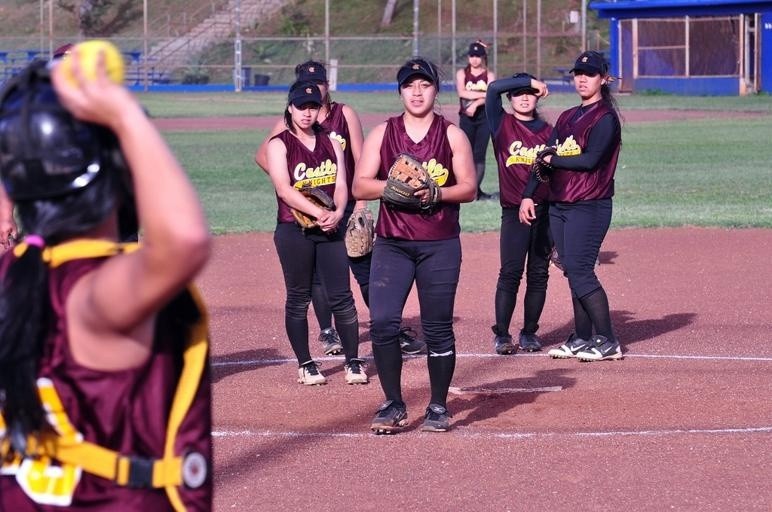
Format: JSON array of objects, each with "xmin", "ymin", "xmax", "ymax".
[{"xmin": 62, "ymin": 39, "xmax": 126, "ymax": 91}]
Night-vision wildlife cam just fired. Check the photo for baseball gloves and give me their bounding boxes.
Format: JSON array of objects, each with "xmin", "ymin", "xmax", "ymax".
[
  {"xmin": 384, "ymin": 155, "xmax": 441, "ymax": 213},
  {"xmin": 345, "ymin": 209, "xmax": 376, "ymax": 257},
  {"xmin": 549, "ymin": 249, "xmax": 563, "ymax": 269},
  {"xmin": 532, "ymin": 147, "xmax": 557, "ymax": 183},
  {"xmin": 291, "ymin": 188, "xmax": 333, "ymax": 229},
  {"xmin": 462, "ymin": 100, "xmax": 476, "ymax": 108}
]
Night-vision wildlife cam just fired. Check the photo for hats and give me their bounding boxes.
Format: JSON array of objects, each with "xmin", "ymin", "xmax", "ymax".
[
  {"xmin": 288, "ymin": 62, "xmax": 326, "ymax": 108},
  {"xmin": 398, "ymin": 60, "xmax": 439, "ymax": 87},
  {"xmin": 509, "ymin": 73, "xmax": 538, "ymax": 96},
  {"xmin": 469, "ymin": 42, "xmax": 486, "ymax": 55},
  {"xmin": 569, "ymin": 50, "xmax": 607, "ymax": 76}
]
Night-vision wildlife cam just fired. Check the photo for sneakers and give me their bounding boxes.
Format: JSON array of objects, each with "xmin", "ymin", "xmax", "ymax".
[
  {"xmin": 492, "ymin": 324, "xmax": 623, "ymax": 362},
  {"xmin": 297, "ymin": 327, "xmax": 452, "ymax": 434}
]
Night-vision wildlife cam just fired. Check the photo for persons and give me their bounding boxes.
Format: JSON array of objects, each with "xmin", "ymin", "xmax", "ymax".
[
  {"xmin": 253, "ymin": 60, "xmax": 428, "ymax": 353},
  {"xmin": 351, "ymin": 55, "xmax": 480, "ymax": 436},
  {"xmin": 0, "ymin": 40, "xmax": 77, "ymax": 252},
  {"xmin": 518, "ymin": 50, "xmax": 633, "ymax": 362},
  {"xmin": 483, "ymin": 69, "xmax": 561, "ymax": 355},
  {"xmin": 266, "ymin": 79, "xmax": 371, "ymax": 384},
  {"xmin": 0, "ymin": 46, "xmax": 213, "ymax": 512},
  {"xmin": 453, "ymin": 40, "xmax": 501, "ymax": 204}
]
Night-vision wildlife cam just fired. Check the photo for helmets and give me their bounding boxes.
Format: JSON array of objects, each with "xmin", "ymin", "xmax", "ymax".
[{"xmin": 0, "ymin": 43, "xmax": 148, "ymax": 201}]
